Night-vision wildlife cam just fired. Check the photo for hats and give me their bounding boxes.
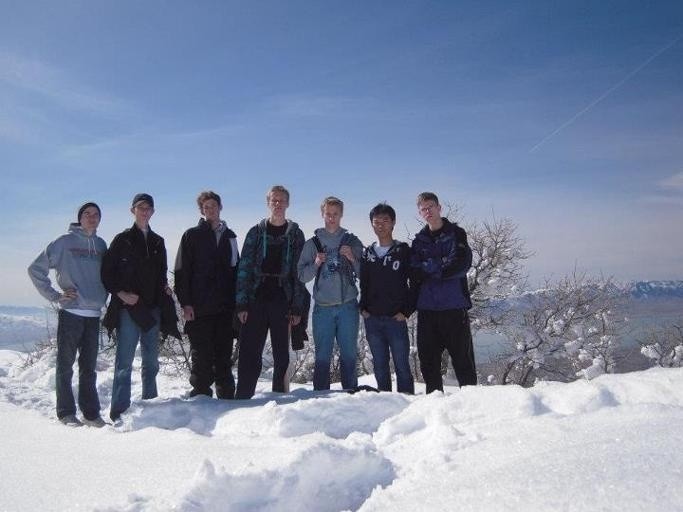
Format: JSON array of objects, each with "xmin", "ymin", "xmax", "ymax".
[
  {"xmin": 77, "ymin": 200, "xmax": 101, "ymax": 222},
  {"xmin": 132, "ymin": 194, "xmax": 154, "ymax": 207}
]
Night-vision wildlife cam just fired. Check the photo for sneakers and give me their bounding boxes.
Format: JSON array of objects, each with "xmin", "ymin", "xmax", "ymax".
[{"xmin": 60, "ymin": 414, "xmax": 123, "ymax": 429}]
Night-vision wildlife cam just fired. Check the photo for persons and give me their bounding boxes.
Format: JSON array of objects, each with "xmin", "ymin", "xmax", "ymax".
[
  {"xmin": 175, "ymin": 192, "xmax": 240, "ymax": 400},
  {"xmin": 102, "ymin": 194, "xmax": 167, "ymax": 421},
  {"xmin": 359, "ymin": 204, "xmax": 417, "ymax": 395},
  {"xmin": 409, "ymin": 192, "xmax": 477, "ymax": 393},
  {"xmin": 298, "ymin": 196, "xmax": 363, "ymax": 389},
  {"xmin": 237, "ymin": 186, "xmax": 305, "ymax": 399},
  {"xmin": 28, "ymin": 202, "xmax": 108, "ymax": 427}
]
